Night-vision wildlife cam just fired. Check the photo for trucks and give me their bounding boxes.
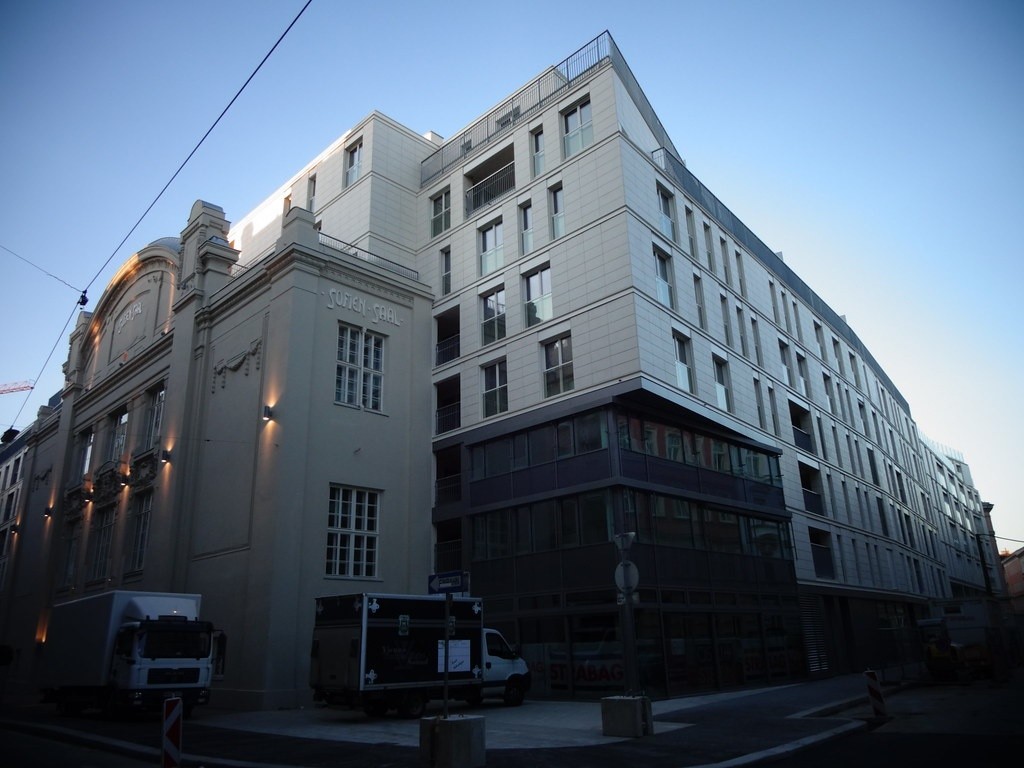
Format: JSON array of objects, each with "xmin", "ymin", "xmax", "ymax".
[
  {"xmin": 310, "ymin": 592, "xmax": 533, "ymax": 720},
  {"xmin": 41, "ymin": 589, "xmax": 227, "ymax": 724}
]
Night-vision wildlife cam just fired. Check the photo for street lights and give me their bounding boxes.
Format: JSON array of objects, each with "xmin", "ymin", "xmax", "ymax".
[{"xmin": 613, "ymin": 529, "xmax": 644, "ymax": 698}]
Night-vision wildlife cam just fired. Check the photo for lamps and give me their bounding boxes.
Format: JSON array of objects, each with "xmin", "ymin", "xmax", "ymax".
[
  {"xmin": 161, "ymin": 448, "xmax": 169, "ymax": 463},
  {"xmin": 263, "ymin": 405, "xmax": 273, "ymax": 421},
  {"xmin": 84, "ymin": 492, "xmax": 90, "ymax": 502},
  {"xmin": 120, "ymin": 475, "xmax": 127, "ymax": 486},
  {"xmin": 45, "ymin": 507, "xmax": 51, "ymax": 517}
]
